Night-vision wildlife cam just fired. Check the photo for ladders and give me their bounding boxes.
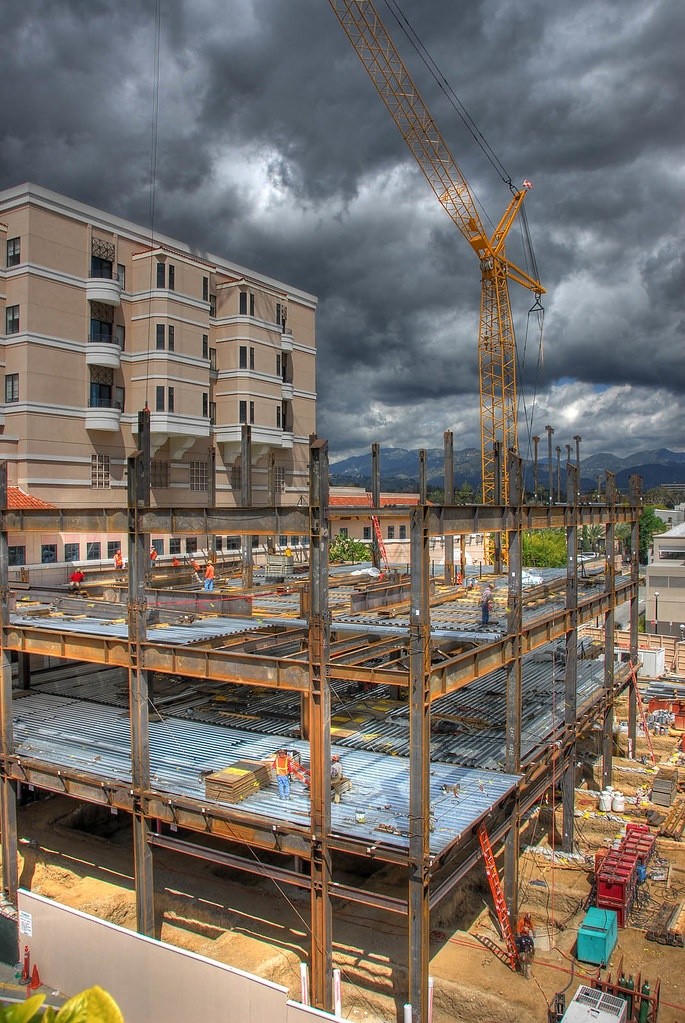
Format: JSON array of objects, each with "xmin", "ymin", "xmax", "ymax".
[
  {"xmin": 367, "ymin": 493, "xmax": 391, "ymax": 573},
  {"xmin": 629, "ymin": 659, "xmax": 655, "ymax": 762},
  {"xmin": 476, "ymin": 823, "xmax": 519, "ymax": 971},
  {"xmin": 288, "ymin": 759, "xmax": 310, "ymax": 787}
]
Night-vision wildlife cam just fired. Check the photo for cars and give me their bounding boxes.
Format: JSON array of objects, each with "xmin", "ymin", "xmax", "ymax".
[{"xmin": 577, "ymin": 551, "xmax": 600, "ymax": 564}]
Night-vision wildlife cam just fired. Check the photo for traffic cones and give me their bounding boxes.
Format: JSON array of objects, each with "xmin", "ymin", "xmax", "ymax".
[{"xmin": 27, "ymin": 964, "xmax": 43, "ymax": 990}]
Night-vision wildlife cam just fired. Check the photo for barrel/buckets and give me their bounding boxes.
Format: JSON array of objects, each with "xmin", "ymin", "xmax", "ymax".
[{"xmin": 599, "ymin": 785, "xmax": 624, "ymax": 813}]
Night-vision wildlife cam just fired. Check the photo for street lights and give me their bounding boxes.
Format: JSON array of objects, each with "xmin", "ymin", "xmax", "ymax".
[
  {"xmin": 680, "ymin": 624, "xmax": 685, "ymax": 642},
  {"xmin": 654, "ymin": 592, "xmax": 659, "ymax": 633}
]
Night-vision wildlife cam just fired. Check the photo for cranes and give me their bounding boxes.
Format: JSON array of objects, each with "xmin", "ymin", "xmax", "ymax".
[{"xmin": 325, "ymin": 1, "xmax": 546, "ymax": 565}]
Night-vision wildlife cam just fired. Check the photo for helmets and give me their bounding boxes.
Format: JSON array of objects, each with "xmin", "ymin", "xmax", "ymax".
[
  {"xmin": 279, "ymin": 750, "xmax": 284, "ymax": 753},
  {"xmin": 330, "ymin": 755, "xmax": 340, "ymax": 761},
  {"xmin": 117, "ymin": 550, "xmax": 121, "ymax": 554},
  {"xmin": 207, "ymin": 560, "xmax": 211, "ymax": 564},
  {"xmin": 191, "ymin": 559, "xmax": 194, "ymax": 561},
  {"xmin": 490, "ymin": 584, "xmax": 495, "ymax": 587},
  {"xmin": 151, "ymin": 547, "xmax": 155, "ymax": 550},
  {"xmin": 76, "ymin": 568, "xmax": 81, "ymax": 572}
]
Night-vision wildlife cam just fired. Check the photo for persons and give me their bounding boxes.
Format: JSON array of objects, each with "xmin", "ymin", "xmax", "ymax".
[
  {"xmin": 190, "ymin": 558, "xmax": 203, "ymax": 581},
  {"xmin": 113, "ymin": 548, "xmax": 123, "ymax": 582},
  {"xmin": 518, "ymin": 912, "xmax": 536, "ymax": 938},
  {"xmin": 273, "ymin": 749, "xmax": 292, "ymax": 801},
  {"xmin": 515, "ymin": 936, "xmax": 535, "ymax": 954},
  {"xmin": 482, "ymin": 583, "xmax": 494, "ymax": 625},
  {"xmin": 150, "ymin": 545, "xmax": 157, "ymax": 566},
  {"xmin": 204, "ymin": 560, "xmax": 214, "ymax": 592},
  {"xmin": 172, "ymin": 557, "xmax": 179, "ymax": 576},
  {"xmin": 68, "ymin": 567, "xmax": 85, "ymax": 590},
  {"xmin": 330, "ymin": 755, "xmax": 342, "ymax": 785}
]
